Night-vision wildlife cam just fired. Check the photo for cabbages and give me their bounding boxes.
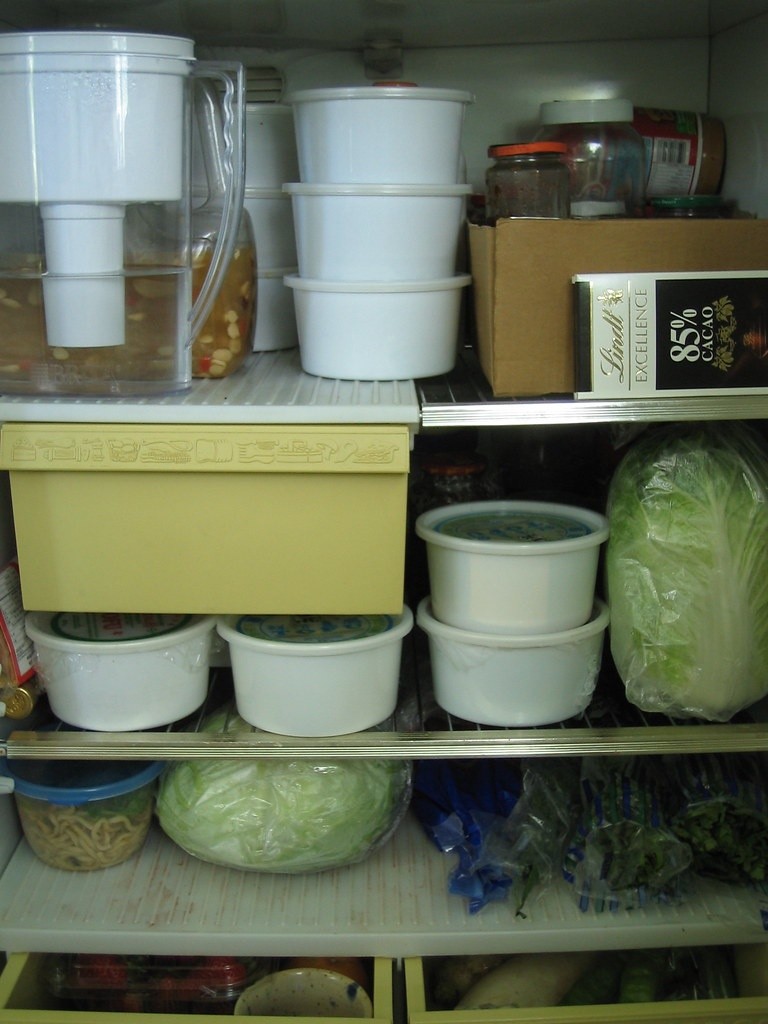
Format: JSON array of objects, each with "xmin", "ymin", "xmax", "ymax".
[
  {"xmin": 151, "ymin": 707, "xmax": 404, "ymax": 874},
  {"xmin": 599, "ymin": 421, "xmax": 768, "ymax": 719}
]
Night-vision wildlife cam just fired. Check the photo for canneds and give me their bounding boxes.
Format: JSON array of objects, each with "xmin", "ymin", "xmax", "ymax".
[{"xmin": 481, "ymin": 95, "xmax": 727, "ymax": 218}]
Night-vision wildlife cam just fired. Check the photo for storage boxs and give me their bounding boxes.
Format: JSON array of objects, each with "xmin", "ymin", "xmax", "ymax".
[
  {"xmin": 37, "ymin": 952, "xmax": 280, "ymax": 1015},
  {"xmin": 464, "ymin": 210, "xmax": 768, "ymax": 397},
  {"xmin": 0, "ymin": 422, "xmax": 410, "ymax": 614}
]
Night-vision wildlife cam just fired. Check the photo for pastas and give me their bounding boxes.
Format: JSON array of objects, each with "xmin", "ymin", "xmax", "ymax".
[{"xmin": 12, "ymin": 791, "xmax": 152, "ymax": 870}]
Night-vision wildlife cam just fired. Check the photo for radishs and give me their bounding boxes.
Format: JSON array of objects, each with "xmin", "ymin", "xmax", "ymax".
[{"xmin": 432, "ymin": 950, "xmax": 602, "ymax": 1011}]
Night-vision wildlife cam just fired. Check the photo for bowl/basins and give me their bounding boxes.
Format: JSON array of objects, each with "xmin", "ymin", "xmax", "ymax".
[
  {"xmin": 285, "ymin": 87, "xmax": 477, "ymax": 184},
  {"xmin": 217, "ymin": 604, "xmax": 414, "ymax": 738},
  {"xmin": 414, "ymin": 500, "xmax": 611, "ymax": 636},
  {"xmin": 258, "ymin": 189, "xmax": 300, "ymax": 269},
  {"xmin": 416, "ymin": 594, "xmax": 611, "ymax": 727},
  {"xmin": 2, "ymin": 759, "xmax": 168, "ymax": 872},
  {"xmin": 281, "ymin": 182, "xmax": 474, "ymax": 280},
  {"xmin": 25, "ymin": 612, "xmax": 217, "ymax": 733},
  {"xmin": 254, "ymin": 269, "xmax": 299, "ymax": 350},
  {"xmin": 234, "ymin": 968, "xmax": 372, "ymax": 1018},
  {"xmin": 284, "ymin": 268, "xmax": 473, "ymax": 381},
  {"xmin": 250, "ymin": 104, "xmax": 301, "ymax": 188}
]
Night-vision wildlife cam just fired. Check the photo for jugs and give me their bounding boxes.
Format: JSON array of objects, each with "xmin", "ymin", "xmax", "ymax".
[
  {"xmin": 0, "ymin": 31, "xmax": 247, "ymax": 399},
  {"xmin": 192, "ymin": 78, "xmax": 259, "ymax": 379}
]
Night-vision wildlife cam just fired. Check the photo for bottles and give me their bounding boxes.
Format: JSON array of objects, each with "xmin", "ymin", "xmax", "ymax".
[
  {"xmin": 530, "ymin": 99, "xmax": 647, "ymax": 218},
  {"xmin": 485, "ymin": 141, "xmax": 572, "ymax": 218},
  {"xmin": 652, "ymin": 196, "xmax": 738, "ymax": 219},
  {"xmin": 633, "ymin": 104, "xmax": 727, "ymax": 196}
]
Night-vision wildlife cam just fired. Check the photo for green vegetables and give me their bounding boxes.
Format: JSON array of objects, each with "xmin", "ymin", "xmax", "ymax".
[{"xmin": 507, "ymin": 753, "xmax": 768, "ymax": 913}]
